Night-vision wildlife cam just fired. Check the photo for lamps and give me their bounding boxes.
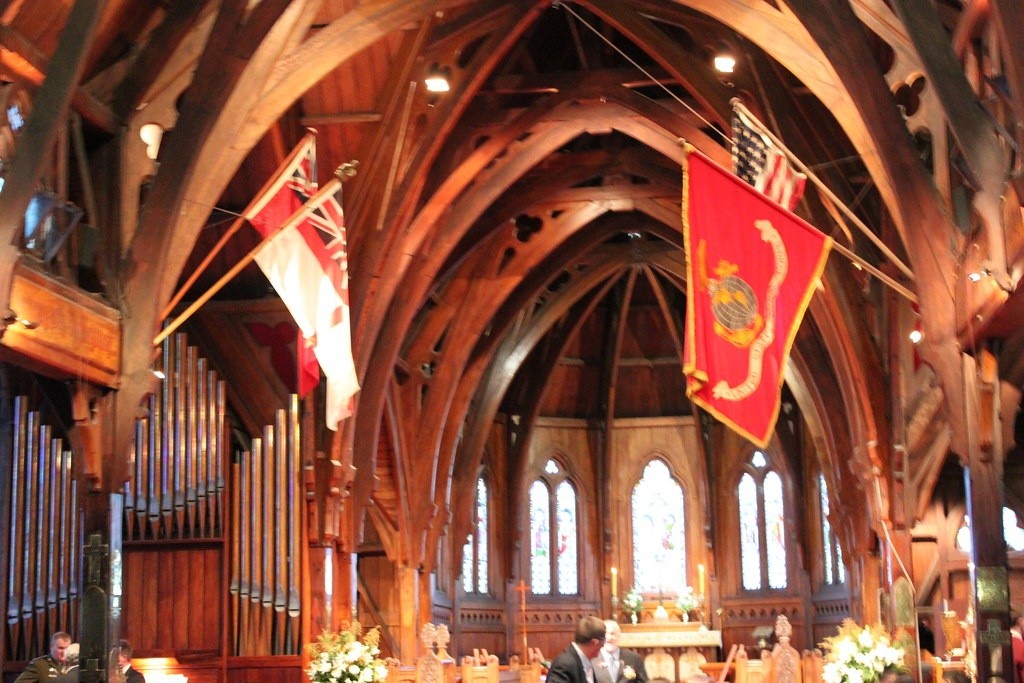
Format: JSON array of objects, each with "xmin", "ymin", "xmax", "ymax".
[
  {"xmin": 713, "ymin": 45, "xmax": 736, "ymax": 72},
  {"xmin": 424, "ymin": 68, "xmax": 450, "ymax": 91}
]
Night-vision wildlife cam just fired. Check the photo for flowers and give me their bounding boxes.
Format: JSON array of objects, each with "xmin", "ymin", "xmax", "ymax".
[
  {"xmin": 303, "ymin": 621, "xmax": 388, "ymax": 683},
  {"xmin": 817, "ymin": 617, "xmax": 905, "ymax": 683}
]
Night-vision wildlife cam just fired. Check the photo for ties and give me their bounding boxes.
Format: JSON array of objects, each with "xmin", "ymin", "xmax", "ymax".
[{"xmin": 610, "ymin": 655, "xmax": 620, "ymax": 683}]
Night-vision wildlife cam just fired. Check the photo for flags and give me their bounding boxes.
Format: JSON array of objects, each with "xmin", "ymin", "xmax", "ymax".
[
  {"xmin": 255, "ymin": 183, "xmax": 361, "ymax": 433},
  {"xmin": 683, "ymin": 146, "xmax": 832, "ymax": 452},
  {"xmin": 247, "ymin": 136, "xmax": 321, "ymax": 399},
  {"xmin": 732, "ymin": 106, "xmax": 807, "ymax": 213}
]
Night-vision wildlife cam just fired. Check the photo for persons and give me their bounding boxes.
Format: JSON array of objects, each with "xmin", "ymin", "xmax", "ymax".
[
  {"xmin": 14, "ymin": 632, "xmax": 71, "ymax": 683},
  {"xmin": 119, "ymin": 639, "xmax": 145, "ymax": 683},
  {"xmin": 591, "ymin": 619, "xmax": 648, "ymax": 683},
  {"xmin": 55, "ymin": 643, "xmax": 80, "ymax": 683},
  {"xmin": 546, "ymin": 616, "xmax": 607, "ymax": 683}
]
